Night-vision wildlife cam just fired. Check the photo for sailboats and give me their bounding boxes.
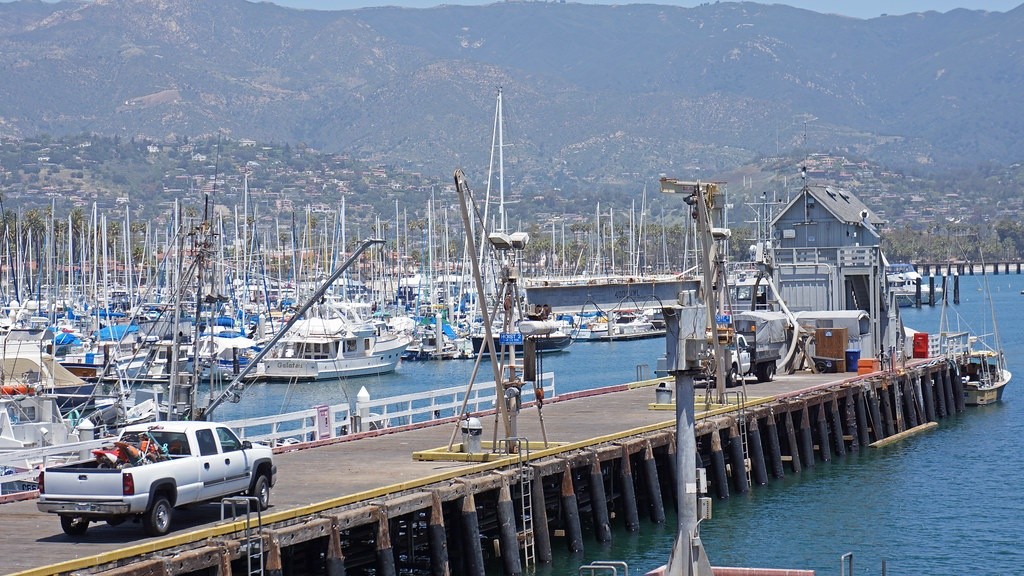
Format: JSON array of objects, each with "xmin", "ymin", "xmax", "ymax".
[
  {"xmin": 0, "ymin": 84, "xmax": 944, "ymax": 490},
  {"xmin": 938, "ymin": 224, "xmax": 1013, "ymax": 407}
]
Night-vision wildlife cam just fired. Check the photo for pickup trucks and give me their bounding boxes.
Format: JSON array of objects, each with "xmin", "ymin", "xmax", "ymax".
[
  {"xmin": 694, "ymin": 311, "xmax": 788, "ymax": 388},
  {"xmin": 32, "ymin": 421, "xmax": 277, "ymax": 537}
]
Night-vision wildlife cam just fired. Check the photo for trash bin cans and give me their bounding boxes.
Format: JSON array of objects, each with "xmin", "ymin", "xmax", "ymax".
[
  {"xmin": 845, "ymin": 348, "xmax": 861, "ymax": 372},
  {"xmin": 655, "ymin": 381, "xmax": 673, "ymax": 404},
  {"xmin": 461, "ymin": 416, "xmax": 483, "ymax": 453},
  {"xmin": 913, "ymin": 333, "xmax": 928, "ymax": 358}
]
